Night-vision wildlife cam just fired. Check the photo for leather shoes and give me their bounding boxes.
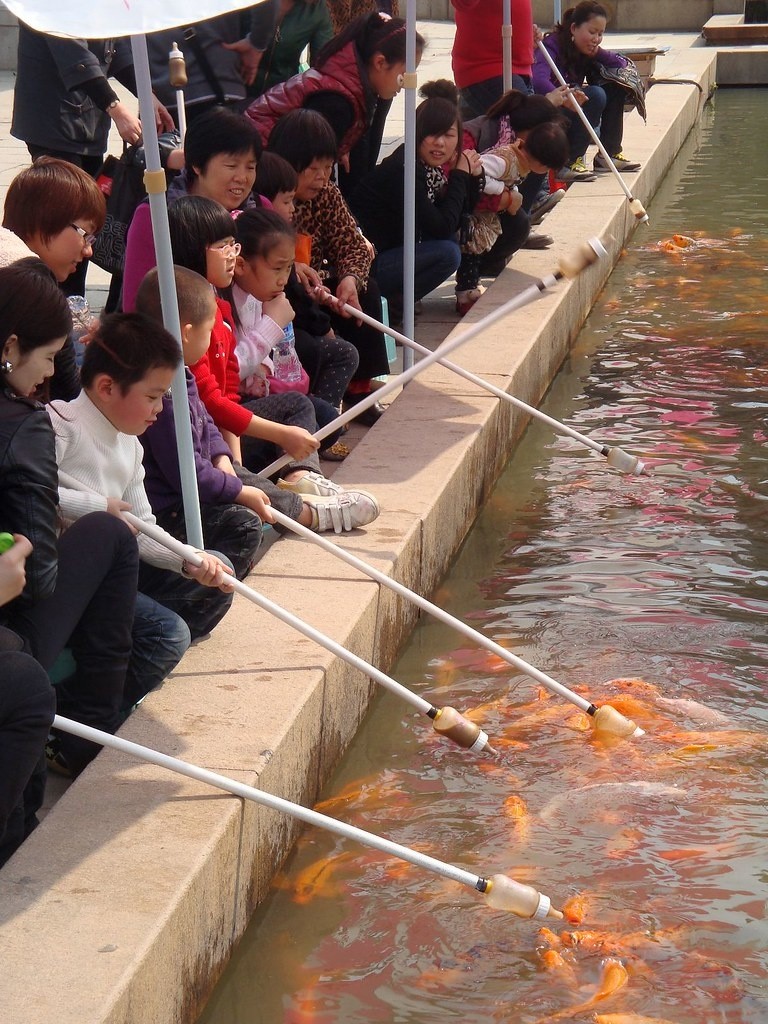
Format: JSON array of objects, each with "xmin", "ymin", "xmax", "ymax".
[{"xmin": 522, "ymin": 230, "xmax": 553, "ymax": 248}]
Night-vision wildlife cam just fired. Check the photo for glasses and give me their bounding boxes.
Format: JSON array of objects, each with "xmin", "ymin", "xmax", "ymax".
[
  {"xmin": 70, "ymin": 222, "xmax": 96, "ymax": 247},
  {"xmin": 206, "ymin": 243, "xmax": 241, "ymax": 258}
]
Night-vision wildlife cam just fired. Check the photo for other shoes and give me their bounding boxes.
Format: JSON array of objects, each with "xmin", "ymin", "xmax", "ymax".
[
  {"xmin": 472, "ymin": 295, "xmax": 481, "ymax": 302},
  {"xmin": 384, "ymin": 296, "xmax": 418, "ymax": 328},
  {"xmin": 319, "ymin": 442, "xmax": 353, "ymax": 461},
  {"xmin": 342, "ymin": 390, "xmax": 381, "ymax": 428},
  {"xmin": 456, "ymin": 301, "xmax": 474, "ymax": 317}
]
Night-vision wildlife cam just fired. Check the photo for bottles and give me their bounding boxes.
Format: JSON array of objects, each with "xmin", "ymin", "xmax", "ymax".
[
  {"xmin": 273, "ymin": 321, "xmax": 301, "ymax": 383},
  {"xmin": 432, "ymin": 706, "xmax": 497, "ymax": 756},
  {"xmin": 606, "ymin": 447, "xmax": 651, "ymax": 477},
  {"xmin": 559, "ymin": 234, "xmax": 615, "ymax": 278},
  {"xmin": 168, "ymin": 42, "xmax": 188, "ymax": 87},
  {"xmin": 629, "ymin": 200, "xmax": 650, "ymax": 226},
  {"xmin": 485, "ymin": 874, "xmax": 564, "ymax": 921},
  {"xmin": 596, "ymin": 704, "xmax": 645, "ymax": 738},
  {"xmin": 68, "ymin": 295, "xmax": 91, "ymax": 371}
]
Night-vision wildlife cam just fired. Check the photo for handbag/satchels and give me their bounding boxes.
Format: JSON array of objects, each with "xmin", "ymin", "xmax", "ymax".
[
  {"xmin": 89, "ymin": 138, "xmax": 173, "ymax": 279},
  {"xmin": 218, "ymin": 96, "xmax": 255, "ymax": 115}
]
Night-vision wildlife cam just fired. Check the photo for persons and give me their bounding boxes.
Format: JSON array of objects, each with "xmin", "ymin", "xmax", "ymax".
[
  {"xmin": 44, "ymin": 312, "xmax": 236, "ymax": 682},
  {"xmin": 0, "ymin": 1, "xmax": 573, "ymax": 868},
  {"xmin": 532, "ymin": 0, "xmax": 647, "ymax": 179}
]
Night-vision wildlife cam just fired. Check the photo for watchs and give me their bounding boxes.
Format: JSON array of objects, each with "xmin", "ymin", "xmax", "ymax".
[{"xmin": 106, "ymin": 100, "xmax": 119, "ymax": 113}]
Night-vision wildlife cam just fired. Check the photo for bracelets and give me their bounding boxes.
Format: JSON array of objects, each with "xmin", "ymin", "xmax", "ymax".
[{"xmin": 506, "ymin": 190, "xmax": 512, "ymax": 209}]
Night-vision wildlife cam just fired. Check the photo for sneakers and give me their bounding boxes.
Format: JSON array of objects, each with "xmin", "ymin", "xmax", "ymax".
[
  {"xmin": 527, "ymin": 189, "xmax": 565, "ymax": 223},
  {"xmin": 276, "ymin": 471, "xmax": 345, "ymax": 496},
  {"xmin": 594, "ymin": 153, "xmax": 642, "ymax": 172},
  {"xmin": 298, "ymin": 488, "xmax": 380, "ymax": 533},
  {"xmin": 553, "ymin": 156, "xmax": 597, "ymax": 182}
]
